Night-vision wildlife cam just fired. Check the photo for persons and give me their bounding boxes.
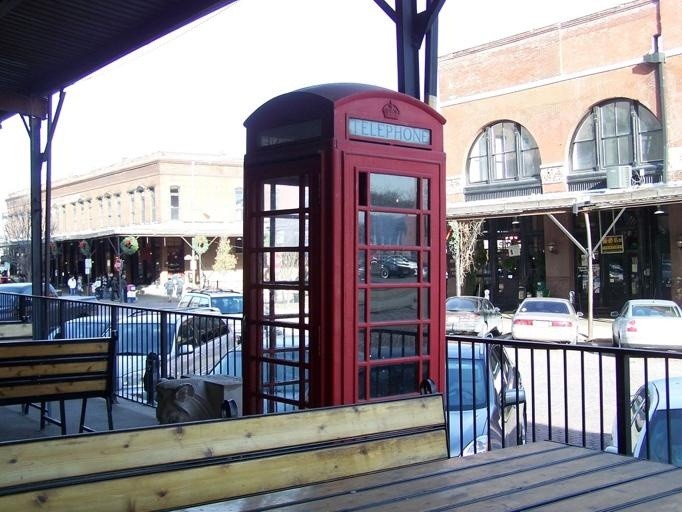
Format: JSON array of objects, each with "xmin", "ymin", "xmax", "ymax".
[
  {"xmin": 133, "ymin": 271, "xmax": 160, "ymax": 285},
  {"xmin": 68, "ymin": 271, "xmax": 136, "ymax": 303},
  {"xmin": 164, "ymin": 277, "xmax": 184, "ymax": 302},
  {"xmin": 0, "ymin": 262, "xmax": 7, "ymax": 275}
]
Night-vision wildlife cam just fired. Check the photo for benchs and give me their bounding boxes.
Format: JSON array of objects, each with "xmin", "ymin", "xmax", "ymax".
[
  {"xmin": 1, "ymin": 320, "xmax": 118, "ymax": 436},
  {"xmin": 1, "ymin": 393, "xmax": 450, "ymax": 512}
]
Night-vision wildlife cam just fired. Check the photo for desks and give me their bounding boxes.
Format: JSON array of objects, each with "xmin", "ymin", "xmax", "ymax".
[{"xmin": 148, "ymin": 439, "xmax": 681, "ymax": 511}]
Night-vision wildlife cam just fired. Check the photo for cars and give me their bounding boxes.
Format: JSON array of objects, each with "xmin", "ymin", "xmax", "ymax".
[
  {"xmin": 612, "ymin": 373, "xmax": 682, "ymax": 465},
  {"xmin": 178, "ymin": 290, "xmax": 244, "ymax": 337},
  {"xmin": 373, "ymin": 253, "xmax": 448, "ymax": 278},
  {"xmin": 0, "ymin": 281, "xmax": 98, "ymax": 333},
  {"xmin": 611, "ymin": 297, "xmax": 682, "ymax": 351},
  {"xmin": 511, "ymin": 296, "xmax": 579, "ymax": 345},
  {"xmin": 445, "ymin": 296, "xmax": 503, "ymax": 338}
]
